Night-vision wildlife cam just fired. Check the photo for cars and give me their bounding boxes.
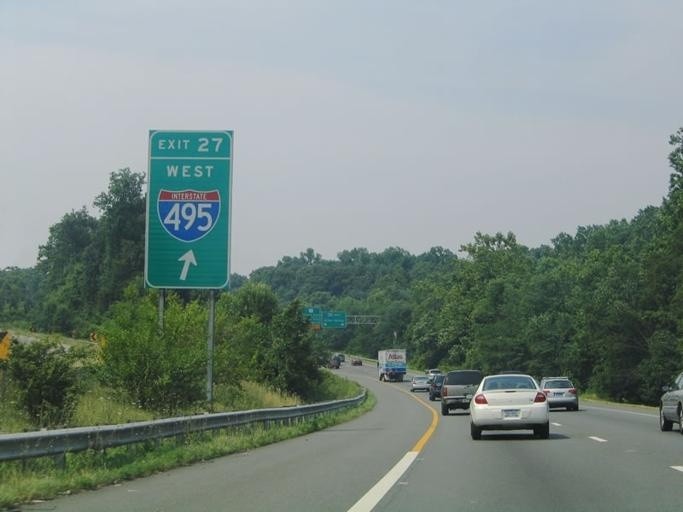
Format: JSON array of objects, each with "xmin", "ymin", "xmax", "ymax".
[
  {"xmin": 411, "ymin": 368, "xmax": 445, "ymax": 402},
  {"xmin": 538, "ymin": 376, "xmax": 579, "ymax": 411},
  {"xmin": 658, "ymin": 371, "xmax": 683, "ymax": 430},
  {"xmin": 467, "ymin": 373, "xmax": 550, "ymax": 441}
]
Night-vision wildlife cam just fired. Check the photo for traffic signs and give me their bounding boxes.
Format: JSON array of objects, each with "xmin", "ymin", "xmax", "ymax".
[{"xmin": 142, "ymin": 127, "xmax": 234, "ymax": 295}]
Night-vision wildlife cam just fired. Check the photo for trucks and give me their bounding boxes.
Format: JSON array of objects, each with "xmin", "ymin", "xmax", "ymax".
[{"xmin": 377, "ymin": 348, "xmax": 408, "ymax": 383}]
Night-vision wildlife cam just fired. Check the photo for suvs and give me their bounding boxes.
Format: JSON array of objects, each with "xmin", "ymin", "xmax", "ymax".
[{"xmin": 440, "ymin": 370, "xmax": 483, "ymax": 416}]
